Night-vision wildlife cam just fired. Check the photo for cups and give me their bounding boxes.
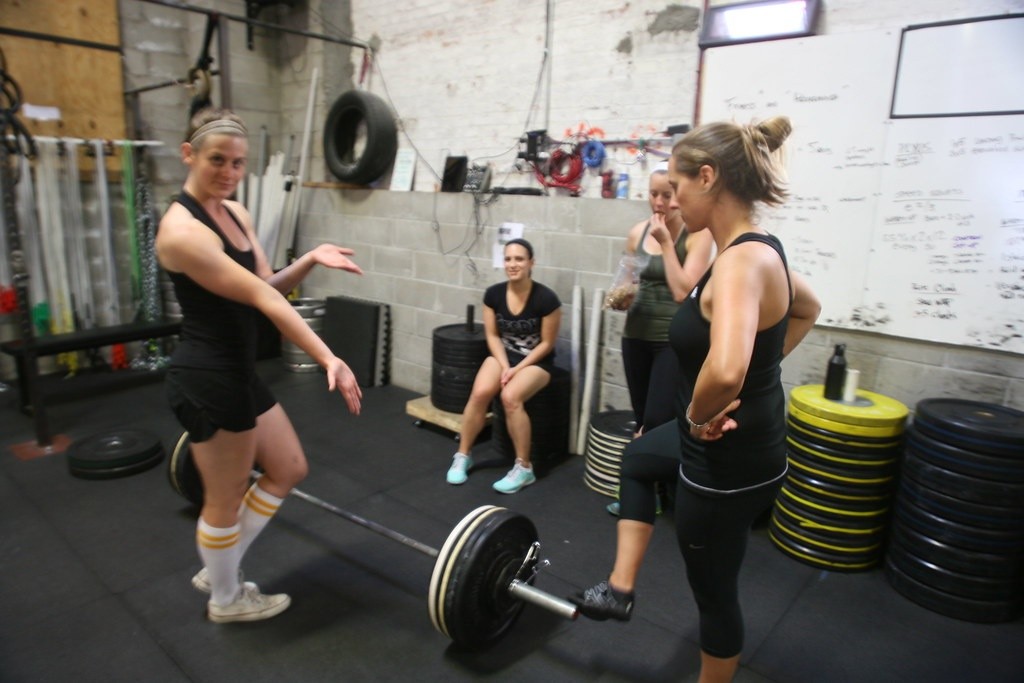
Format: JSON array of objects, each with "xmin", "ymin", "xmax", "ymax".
[{"xmin": 843, "ymin": 369, "xmax": 861, "ymax": 402}]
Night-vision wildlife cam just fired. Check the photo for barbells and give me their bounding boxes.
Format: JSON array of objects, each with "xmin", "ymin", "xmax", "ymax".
[{"xmin": 165, "ymin": 429, "xmax": 579, "ymax": 646}]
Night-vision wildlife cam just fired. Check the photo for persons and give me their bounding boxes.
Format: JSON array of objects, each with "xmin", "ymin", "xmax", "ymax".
[
  {"xmin": 608, "ymin": 159, "xmax": 717, "ymax": 518},
  {"xmin": 567, "ymin": 118, "xmax": 821, "ymax": 683},
  {"xmin": 155, "ymin": 109, "xmax": 362, "ymax": 623},
  {"xmin": 445, "ymin": 238, "xmax": 561, "ymax": 493}
]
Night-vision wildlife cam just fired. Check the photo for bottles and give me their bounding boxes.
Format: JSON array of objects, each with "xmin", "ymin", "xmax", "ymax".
[
  {"xmin": 616, "ymin": 170, "xmax": 629, "ymax": 199},
  {"xmin": 823, "ymin": 343, "xmax": 847, "ymax": 400}
]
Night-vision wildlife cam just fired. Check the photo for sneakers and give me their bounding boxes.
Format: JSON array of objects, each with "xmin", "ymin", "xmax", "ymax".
[
  {"xmin": 492, "ymin": 458, "xmax": 537, "ymax": 494},
  {"xmin": 569, "ymin": 578, "xmax": 635, "ymax": 622},
  {"xmin": 194, "ymin": 567, "xmax": 262, "ymax": 596},
  {"xmin": 445, "ymin": 452, "xmax": 474, "ymax": 485},
  {"xmin": 608, "ymin": 491, "xmax": 664, "ymax": 518},
  {"xmin": 207, "ymin": 583, "xmax": 293, "ymax": 624}
]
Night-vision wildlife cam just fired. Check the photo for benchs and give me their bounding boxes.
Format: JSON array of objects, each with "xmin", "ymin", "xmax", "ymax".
[{"xmin": 0, "ymin": 317, "xmax": 182, "ymax": 448}]
